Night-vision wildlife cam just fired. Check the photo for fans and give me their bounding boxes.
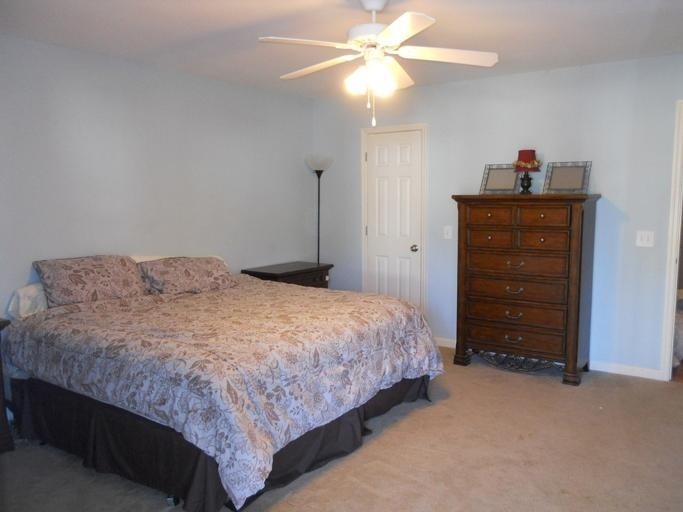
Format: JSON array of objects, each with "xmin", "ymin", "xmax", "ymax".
[{"xmin": 258, "ymin": 0, "xmax": 498, "ymax": 90}]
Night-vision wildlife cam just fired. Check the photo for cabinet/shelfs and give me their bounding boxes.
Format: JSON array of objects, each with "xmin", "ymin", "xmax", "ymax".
[{"xmin": 451, "ymin": 194, "xmax": 602, "ymax": 387}]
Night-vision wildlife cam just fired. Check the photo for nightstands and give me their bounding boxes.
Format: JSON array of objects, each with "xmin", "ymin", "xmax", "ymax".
[{"xmin": 241, "ymin": 261, "xmax": 334, "ymax": 288}]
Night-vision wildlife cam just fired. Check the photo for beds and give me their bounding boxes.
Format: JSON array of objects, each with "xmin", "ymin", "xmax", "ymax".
[{"xmin": 0, "ymin": 272, "xmax": 447, "ymax": 511}]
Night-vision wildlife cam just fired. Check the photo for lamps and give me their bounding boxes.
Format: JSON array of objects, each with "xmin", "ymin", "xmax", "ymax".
[
  {"xmin": 513, "ymin": 149, "xmax": 543, "ymax": 196},
  {"xmin": 304, "ymin": 149, "xmax": 332, "ymax": 263},
  {"xmin": 345, "ymin": 48, "xmax": 397, "ymax": 127}
]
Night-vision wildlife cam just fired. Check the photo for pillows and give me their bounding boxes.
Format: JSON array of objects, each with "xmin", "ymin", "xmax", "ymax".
[
  {"xmin": 137, "ymin": 256, "xmax": 239, "ymax": 294},
  {"xmin": 32, "ymin": 254, "xmax": 152, "ymax": 309}
]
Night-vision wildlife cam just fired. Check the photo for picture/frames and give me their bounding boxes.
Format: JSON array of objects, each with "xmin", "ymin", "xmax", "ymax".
[
  {"xmin": 479, "ymin": 164, "xmax": 521, "ymax": 195},
  {"xmin": 543, "ymin": 161, "xmax": 592, "ymax": 194}
]
